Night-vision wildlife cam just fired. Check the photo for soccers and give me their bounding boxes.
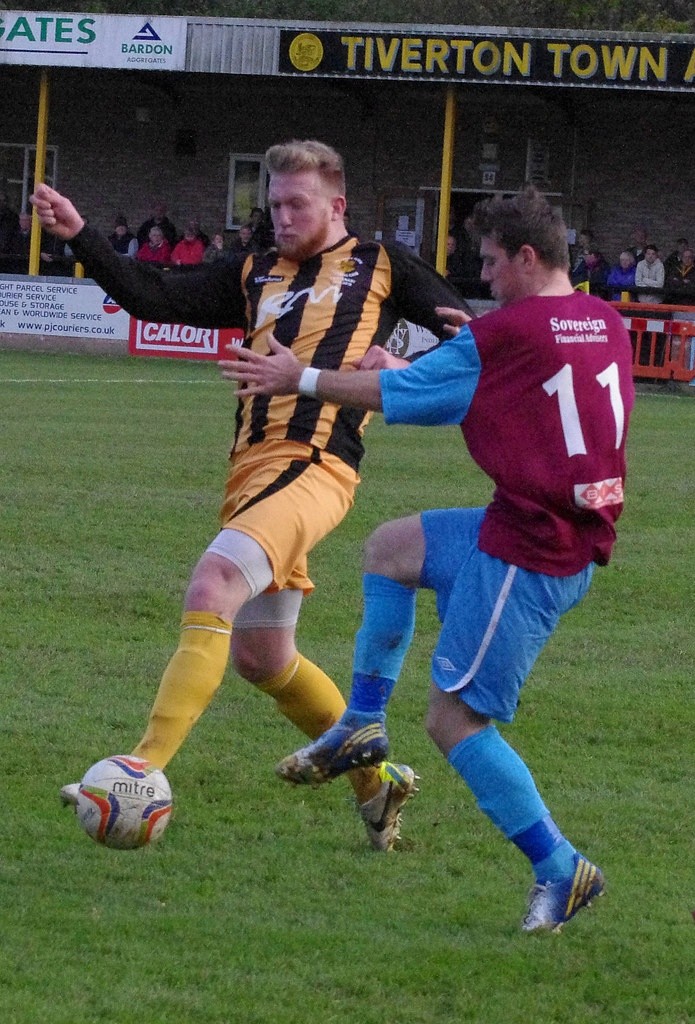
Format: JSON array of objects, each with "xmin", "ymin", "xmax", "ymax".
[{"xmin": 76, "ymin": 754, "xmax": 173, "ymax": 851}]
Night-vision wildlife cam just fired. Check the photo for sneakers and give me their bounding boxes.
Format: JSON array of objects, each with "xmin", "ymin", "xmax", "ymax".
[
  {"xmin": 522, "ymin": 852, "xmax": 606, "ymax": 933},
  {"xmin": 59, "ymin": 782, "xmax": 80, "ymax": 805},
  {"xmin": 359, "ymin": 760, "xmax": 420, "ymax": 854},
  {"xmin": 277, "ymin": 709, "xmax": 390, "ymax": 782}
]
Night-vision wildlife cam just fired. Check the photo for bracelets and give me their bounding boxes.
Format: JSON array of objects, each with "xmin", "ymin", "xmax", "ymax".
[{"xmin": 300, "ymin": 368, "xmax": 319, "ymax": 401}]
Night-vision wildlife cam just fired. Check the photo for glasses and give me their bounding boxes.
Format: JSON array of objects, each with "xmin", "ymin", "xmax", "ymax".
[{"xmin": 0, "ymin": 199, "xmax": 7, "ymax": 202}]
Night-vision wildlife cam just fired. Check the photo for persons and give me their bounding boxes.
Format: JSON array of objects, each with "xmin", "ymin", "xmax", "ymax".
[
  {"xmin": 29, "ymin": 138, "xmax": 481, "ymax": 849},
  {"xmin": 341, "ymin": 208, "xmax": 695, "ymax": 386},
  {"xmin": 1, "ymin": 206, "xmax": 264, "ymax": 278},
  {"xmin": 215, "ymin": 188, "xmax": 637, "ymax": 932}
]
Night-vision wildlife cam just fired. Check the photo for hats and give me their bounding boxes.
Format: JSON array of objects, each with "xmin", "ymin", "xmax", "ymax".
[{"xmin": 115, "ymin": 216, "xmax": 127, "ymax": 229}]
